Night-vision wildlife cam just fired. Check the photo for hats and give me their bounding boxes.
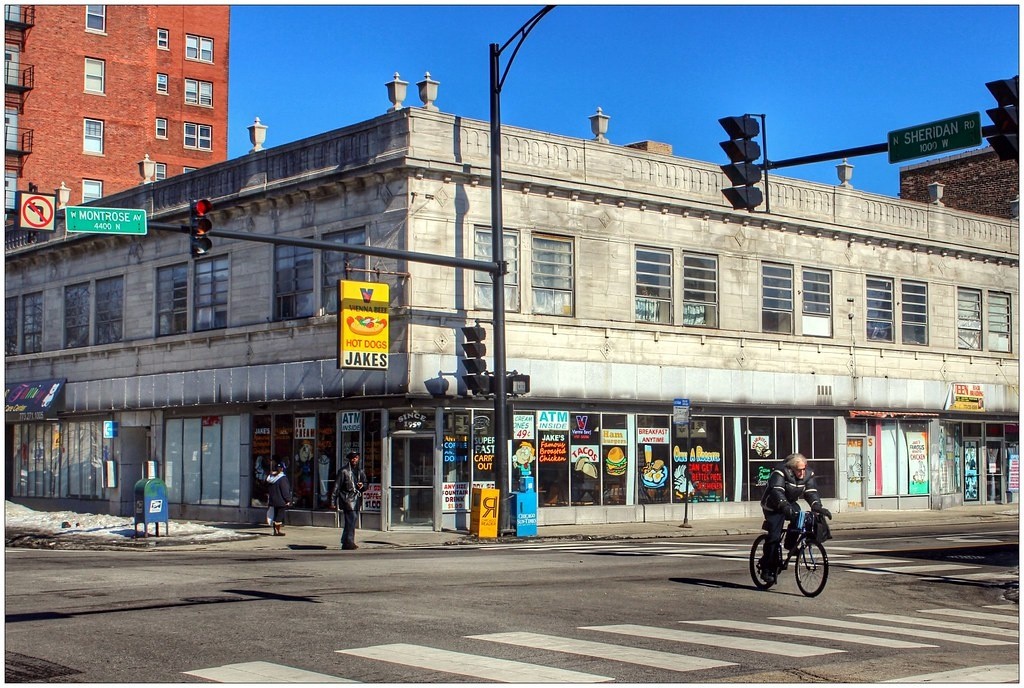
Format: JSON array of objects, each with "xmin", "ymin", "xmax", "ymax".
[
  {"xmin": 275, "ymin": 462, "xmax": 287, "ymax": 468},
  {"xmin": 346, "ymin": 451, "xmax": 360, "ymax": 459}
]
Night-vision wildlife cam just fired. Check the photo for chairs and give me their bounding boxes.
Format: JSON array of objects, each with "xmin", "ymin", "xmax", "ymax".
[{"xmin": 542, "ymin": 484, "xmax": 723, "ymax": 506}]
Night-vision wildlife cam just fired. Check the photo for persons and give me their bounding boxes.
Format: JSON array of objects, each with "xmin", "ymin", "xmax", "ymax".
[
  {"xmin": 331, "ymin": 451, "xmax": 369, "ymax": 550},
  {"xmin": 761, "ymin": 453, "xmax": 826, "ymax": 583},
  {"xmin": 266, "ymin": 463, "xmax": 291, "ymax": 536}
]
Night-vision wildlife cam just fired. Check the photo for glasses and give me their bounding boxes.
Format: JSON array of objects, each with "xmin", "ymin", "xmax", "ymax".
[{"xmin": 793, "ymin": 467, "xmax": 806, "ymax": 471}]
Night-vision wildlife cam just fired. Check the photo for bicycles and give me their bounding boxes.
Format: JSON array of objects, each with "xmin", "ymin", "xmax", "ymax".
[{"xmin": 749, "ymin": 508, "xmax": 829, "ymax": 597}]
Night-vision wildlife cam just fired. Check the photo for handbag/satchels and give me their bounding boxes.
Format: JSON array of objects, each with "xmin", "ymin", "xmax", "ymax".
[
  {"xmin": 267, "ymin": 506, "xmax": 274, "ymax": 526},
  {"xmin": 806, "ymin": 513, "xmax": 832, "ymax": 543}
]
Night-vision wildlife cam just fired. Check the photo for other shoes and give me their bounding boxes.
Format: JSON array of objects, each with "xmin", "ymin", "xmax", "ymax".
[
  {"xmin": 760, "ymin": 570, "xmax": 774, "ymax": 582},
  {"xmin": 784, "ymin": 543, "xmax": 800, "ymax": 554},
  {"xmin": 342, "ymin": 543, "xmax": 358, "ymax": 550}
]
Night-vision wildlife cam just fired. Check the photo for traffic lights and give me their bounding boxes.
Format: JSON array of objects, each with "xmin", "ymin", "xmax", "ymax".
[
  {"xmin": 462, "ymin": 326, "xmax": 491, "ymax": 395},
  {"xmin": 190, "ymin": 199, "xmax": 213, "ymax": 260},
  {"xmin": 986, "ymin": 75, "xmax": 1019, "ymax": 162},
  {"xmin": 720, "ymin": 114, "xmax": 765, "ymax": 209}
]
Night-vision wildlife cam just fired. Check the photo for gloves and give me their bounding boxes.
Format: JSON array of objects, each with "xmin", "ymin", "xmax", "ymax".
[
  {"xmin": 812, "ymin": 502, "xmax": 831, "ymax": 520},
  {"xmin": 790, "ymin": 508, "xmax": 811, "ymax": 523}
]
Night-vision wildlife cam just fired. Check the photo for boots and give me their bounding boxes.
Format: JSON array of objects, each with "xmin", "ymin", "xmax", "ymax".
[{"xmin": 273, "ymin": 521, "xmax": 285, "ymax": 536}]
